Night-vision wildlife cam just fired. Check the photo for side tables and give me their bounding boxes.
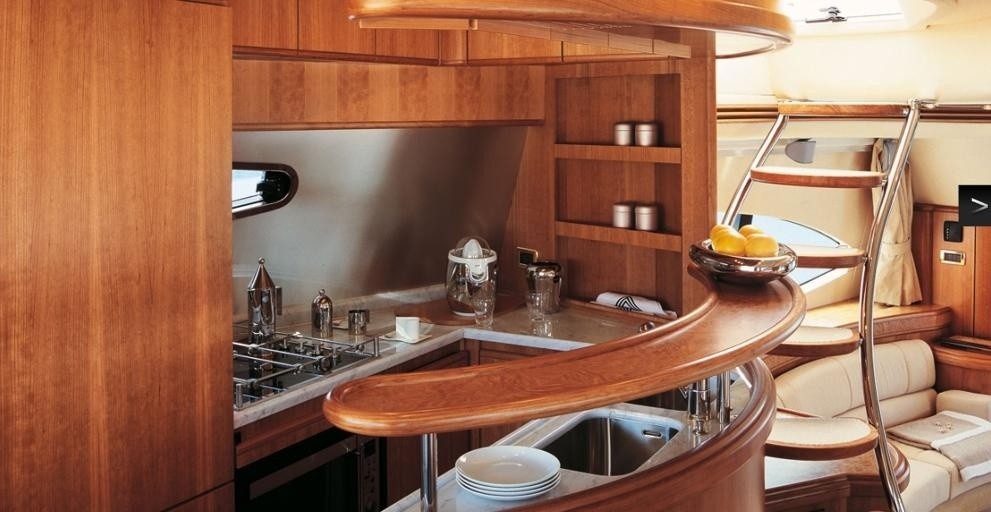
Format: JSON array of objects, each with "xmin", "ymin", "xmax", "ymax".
[{"xmin": 762, "ymin": 442, "xmax": 911, "ymax": 511}]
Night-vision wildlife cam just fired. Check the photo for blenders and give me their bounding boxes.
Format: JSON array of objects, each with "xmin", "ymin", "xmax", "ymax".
[{"xmin": 446, "ymin": 237, "xmax": 501, "ymax": 315}]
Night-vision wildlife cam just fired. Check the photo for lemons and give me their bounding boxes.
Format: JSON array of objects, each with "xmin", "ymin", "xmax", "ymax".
[{"xmin": 709, "ymin": 224, "xmax": 779, "ymax": 257}]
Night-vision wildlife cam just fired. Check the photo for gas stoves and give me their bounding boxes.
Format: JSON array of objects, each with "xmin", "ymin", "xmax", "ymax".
[{"xmin": 230, "ymin": 334, "xmax": 382, "ymax": 412}]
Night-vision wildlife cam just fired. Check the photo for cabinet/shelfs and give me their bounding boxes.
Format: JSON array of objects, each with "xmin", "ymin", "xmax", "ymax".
[{"xmin": 554, "ymin": 143, "xmax": 681, "ymax": 253}]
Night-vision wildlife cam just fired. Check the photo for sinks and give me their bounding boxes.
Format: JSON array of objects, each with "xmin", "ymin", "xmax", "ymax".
[
  {"xmin": 623, "ymin": 369, "xmax": 741, "ymax": 409},
  {"xmin": 530, "ymin": 409, "xmax": 686, "ymax": 476}
]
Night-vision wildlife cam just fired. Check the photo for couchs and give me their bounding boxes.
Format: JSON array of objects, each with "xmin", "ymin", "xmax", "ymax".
[{"xmin": 774, "ymin": 340, "xmax": 991, "ymax": 512}]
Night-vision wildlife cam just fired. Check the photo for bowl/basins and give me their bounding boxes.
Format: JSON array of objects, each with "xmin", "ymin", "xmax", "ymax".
[{"xmin": 688, "ymin": 236, "xmax": 799, "ymax": 290}]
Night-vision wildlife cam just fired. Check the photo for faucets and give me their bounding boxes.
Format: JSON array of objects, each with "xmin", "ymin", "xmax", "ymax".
[{"xmin": 637, "ymin": 321, "xmax": 697, "ymax": 420}]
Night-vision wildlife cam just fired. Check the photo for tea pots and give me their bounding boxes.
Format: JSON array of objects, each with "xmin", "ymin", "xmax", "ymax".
[{"xmin": 525, "ymin": 262, "xmax": 563, "ymax": 313}]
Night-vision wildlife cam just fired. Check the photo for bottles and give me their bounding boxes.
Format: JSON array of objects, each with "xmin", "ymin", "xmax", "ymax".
[
  {"xmin": 311, "ymin": 288, "xmax": 334, "ymax": 337},
  {"xmin": 613, "ymin": 203, "xmax": 635, "ymax": 228},
  {"xmin": 635, "ymin": 123, "xmax": 659, "ymax": 147},
  {"xmin": 247, "ymin": 257, "xmax": 284, "ymax": 334},
  {"xmin": 614, "ymin": 122, "xmax": 634, "ymax": 146},
  {"xmin": 634, "ymin": 205, "xmax": 658, "ymax": 231}
]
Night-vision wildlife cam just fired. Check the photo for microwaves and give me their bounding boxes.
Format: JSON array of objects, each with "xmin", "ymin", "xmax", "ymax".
[{"xmin": 236, "ymin": 434, "xmax": 383, "ymax": 512}]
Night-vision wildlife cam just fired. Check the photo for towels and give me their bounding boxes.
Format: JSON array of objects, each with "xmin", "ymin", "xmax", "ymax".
[{"xmin": 885, "ymin": 410, "xmax": 991, "ymax": 482}]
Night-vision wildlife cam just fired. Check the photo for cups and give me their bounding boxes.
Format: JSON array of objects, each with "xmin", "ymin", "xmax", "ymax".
[
  {"xmin": 524, "ymin": 288, "xmax": 551, "ymax": 320},
  {"xmin": 347, "ymin": 308, "xmax": 371, "ymax": 335},
  {"xmin": 472, "ymin": 297, "xmax": 496, "ymax": 327},
  {"xmin": 531, "ymin": 319, "xmax": 556, "ymax": 338},
  {"xmin": 397, "ymin": 315, "xmax": 420, "ymax": 337}
]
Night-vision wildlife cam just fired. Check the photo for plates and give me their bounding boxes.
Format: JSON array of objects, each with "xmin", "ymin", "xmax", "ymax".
[
  {"xmin": 454, "ymin": 446, "xmax": 563, "ymax": 500},
  {"xmin": 385, "ymin": 337, "xmax": 434, "ymax": 343}
]
